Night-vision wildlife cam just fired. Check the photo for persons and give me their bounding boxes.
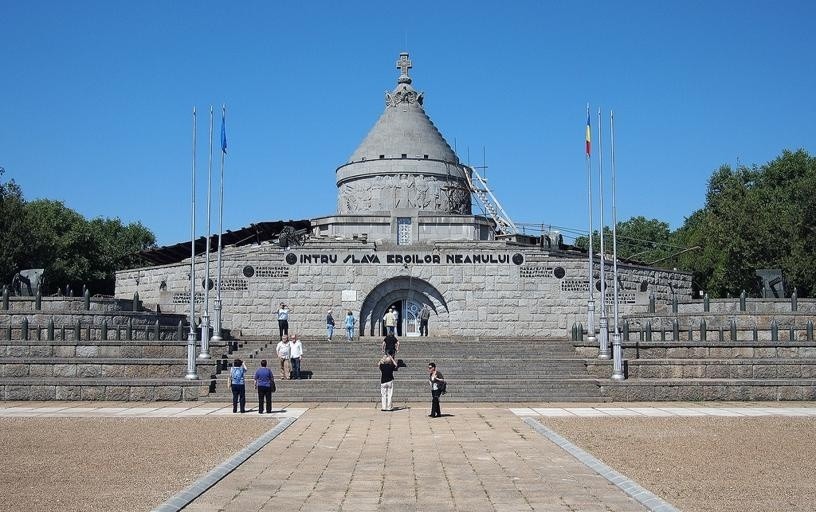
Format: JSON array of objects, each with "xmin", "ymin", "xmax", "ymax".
[{"xmin": 229, "ymin": 301, "xmax": 446, "ymax": 418}]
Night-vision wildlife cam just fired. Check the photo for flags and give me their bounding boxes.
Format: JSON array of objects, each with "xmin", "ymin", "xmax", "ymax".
[
  {"xmin": 584, "ymin": 109, "xmax": 592, "ymax": 158},
  {"xmin": 219, "ymin": 109, "xmax": 229, "ymax": 155}
]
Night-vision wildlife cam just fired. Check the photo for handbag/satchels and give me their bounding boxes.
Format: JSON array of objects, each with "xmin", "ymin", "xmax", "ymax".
[
  {"xmin": 270, "ymin": 381, "xmax": 276, "ymax": 392},
  {"xmin": 437, "ymin": 383, "xmax": 446, "ymax": 391}
]
